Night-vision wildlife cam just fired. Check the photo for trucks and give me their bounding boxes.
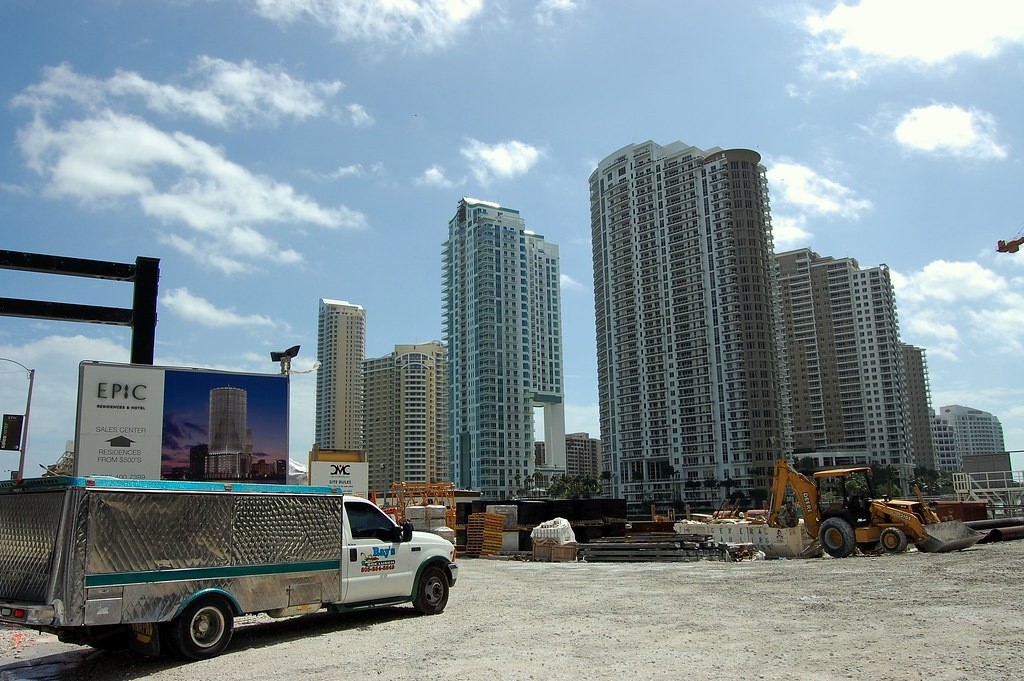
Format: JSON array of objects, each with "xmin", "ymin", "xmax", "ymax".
[{"xmin": 0, "ymin": 473, "xmax": 459, "ymax": 659}]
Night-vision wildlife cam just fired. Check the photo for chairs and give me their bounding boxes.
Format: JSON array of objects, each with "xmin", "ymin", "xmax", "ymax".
[{"xmin": 848, "ymin": 495, "xmax": 861, "ymax": 509}]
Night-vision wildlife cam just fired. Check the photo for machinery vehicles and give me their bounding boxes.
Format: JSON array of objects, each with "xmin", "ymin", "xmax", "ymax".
[
  {"xmin": 367, "ymin": 491, "xmax": 398, "ymax": 525},
  {"xmin": 769, "ymin": 459, "xmax": 987, "ymax": 560}
]
